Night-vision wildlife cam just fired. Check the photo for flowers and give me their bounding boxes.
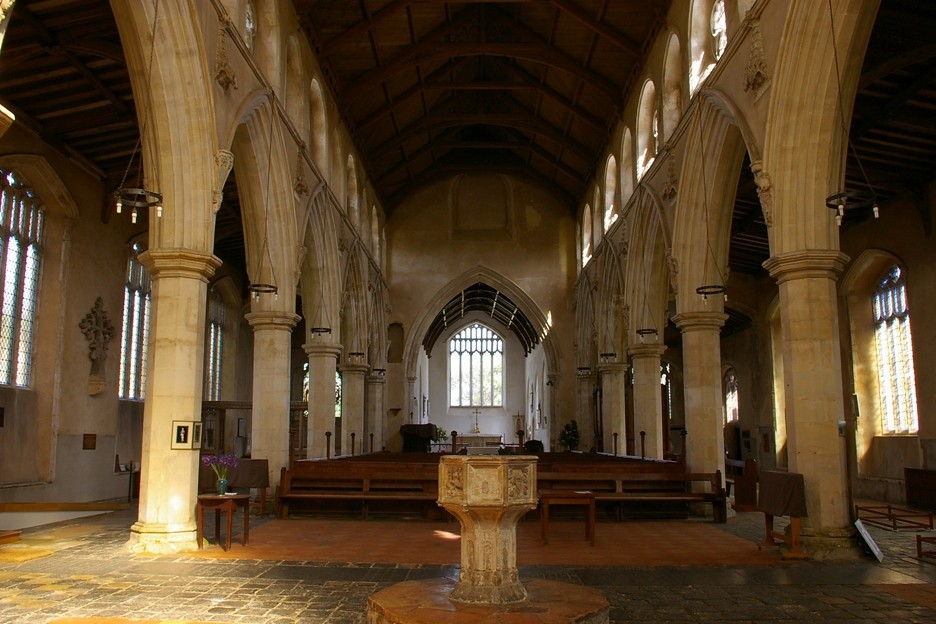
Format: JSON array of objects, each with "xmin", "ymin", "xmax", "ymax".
[
  {"xmin": 432, "ymin": 426, "xmax": 448, "ymax": 441},
  {"xmin": 558, "ymin": 423, "xmax": 580, "ymax": 444},
  {"xmin": 200, "ymin": 453, "xmax": 238, "ymax": 477}
]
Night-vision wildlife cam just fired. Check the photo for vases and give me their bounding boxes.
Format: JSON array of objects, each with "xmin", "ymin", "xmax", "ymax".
[
  {"xmin": 567, "ymin": 440, "xmax": 575, "ymax": 450},
  {"xmin": 438, "ymin": 439, "xmax": 442, "ymax": 449},
  {"xmin": 216, "ymin": 475, "xmax": 229, "ymax": 493}
]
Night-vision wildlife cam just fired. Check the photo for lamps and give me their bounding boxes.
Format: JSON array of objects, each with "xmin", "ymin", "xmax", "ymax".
[
  {"xmin": 575, "ymin": 0, "xmax": 890, "ymax": 386},
  {"xmin": 113, "ymin": 0, "xmax": 388, "ymax": 383}
]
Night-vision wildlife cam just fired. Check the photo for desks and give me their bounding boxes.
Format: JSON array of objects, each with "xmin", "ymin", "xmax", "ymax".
[
  {"xmin": 198, "ymin": 493, "xmax": 251, "ymax": 552},
  {"xmin": 538, "ymin": 490, "xmax": 594, "ymax": 540}
]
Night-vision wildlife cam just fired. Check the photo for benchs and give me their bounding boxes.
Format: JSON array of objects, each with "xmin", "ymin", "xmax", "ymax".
[
  {"xmin": 274, "ymin": 449, "xmax": 470, "ymax": 520},
  {"xmin": 503, "ymin": 446, "xmax": 730, "ymax": 523}
]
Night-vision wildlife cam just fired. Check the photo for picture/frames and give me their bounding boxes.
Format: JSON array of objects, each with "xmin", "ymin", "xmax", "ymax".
[
  {"xmin": 171, "ymin": 420, "xmax": 194, "ymax": 450},
  {"xmin": 192, "ymin": 422, "xmax": 202, "ymax": 449},
  {"xmin": 238, "ymin": 418, "xmax": 246, "ymax": 437}
]
